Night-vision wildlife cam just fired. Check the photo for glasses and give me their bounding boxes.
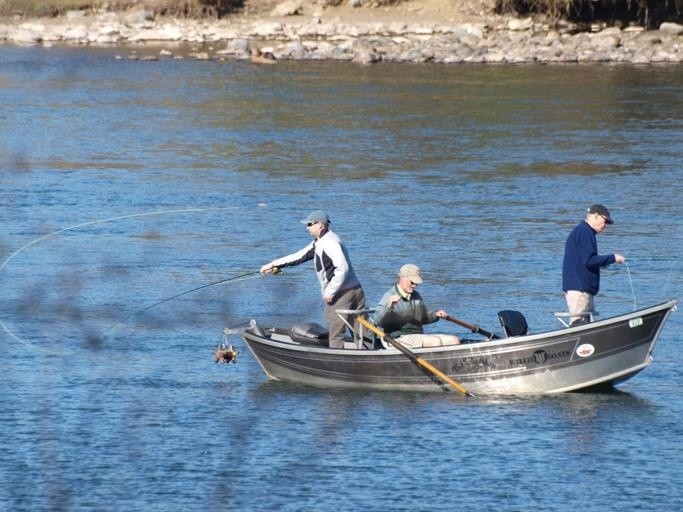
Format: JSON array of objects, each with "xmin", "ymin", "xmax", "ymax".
[
  {"xmin": 308, "ymin": 222, "xmax": 318, "ymax": 226},
  {"xmin": 601, "ymin": 215, "xmax": 608, "ymax": 225},
  {"xmin": 407, "ymin": 280, "xmax": 415, "ymax": 285}
]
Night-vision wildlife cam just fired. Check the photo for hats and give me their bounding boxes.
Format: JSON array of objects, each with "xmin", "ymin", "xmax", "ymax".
[
  {"xmin": 587, "ymin": 204, "xmax": 614, "ymax": 225},
  {"xmin": 300, "ymin": 210, "xmax": 328, "ymax": 224},
  {"xmin": 400, "ymin": 265, "xmax": 423, "ymax": 284}
]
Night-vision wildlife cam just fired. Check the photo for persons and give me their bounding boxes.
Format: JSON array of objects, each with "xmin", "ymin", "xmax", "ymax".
[
  {"xmin": 562, "ymin": 203, "xmax": 626, "ymax": 325},
  {"xmin": 259, "ymin": 210, "xmax": 376, "ymax": 349},
  {"xmin": 370, "ymin": 264, "xmax": 461, "ymax": 348}
]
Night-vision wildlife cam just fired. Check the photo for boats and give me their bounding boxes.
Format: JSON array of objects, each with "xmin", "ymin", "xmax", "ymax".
[{"xmin": 223, "ymin": 300, "xmax": 679, "ymax": 398}]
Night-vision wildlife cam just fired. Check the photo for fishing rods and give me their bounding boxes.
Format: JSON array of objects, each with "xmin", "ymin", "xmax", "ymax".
[
  {"xmin": 103, "ymin": 270, "xmax": 284, "ymax": 332},
  {"xmin": 624, "ymin": 256, "xmax": 678, "ymax": 261}
]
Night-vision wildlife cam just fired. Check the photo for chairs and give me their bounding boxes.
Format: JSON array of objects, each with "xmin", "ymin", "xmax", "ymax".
[{"xmin": 498, "ymin": 310, "xmax": 532, "ymax": 337}]
